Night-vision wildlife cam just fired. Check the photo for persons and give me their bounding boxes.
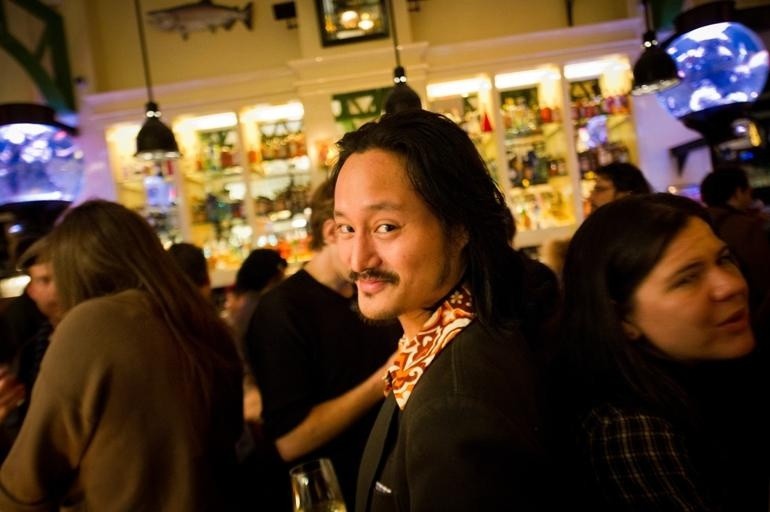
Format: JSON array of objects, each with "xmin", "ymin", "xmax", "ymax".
[{"xmin": 1, "ymin": 111, "xmax": 769, "ymax": 511}]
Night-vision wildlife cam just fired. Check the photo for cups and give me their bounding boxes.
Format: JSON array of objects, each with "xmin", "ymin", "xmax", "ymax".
[{"xmin": 289, "ymin": 456, "xmax": 345, "ymax": 511}]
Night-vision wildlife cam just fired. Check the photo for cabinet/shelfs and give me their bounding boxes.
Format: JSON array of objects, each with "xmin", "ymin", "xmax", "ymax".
[{"xmin": 87, "ymin": 22, "xmax": 647, "ymax": 296}]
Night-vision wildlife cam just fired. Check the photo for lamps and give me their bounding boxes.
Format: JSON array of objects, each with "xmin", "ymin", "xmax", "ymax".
[
  {"xmin": 132, "ymin": 0, "xmax": 180, "ymax": 159},
  {"xmin": 629, "ymin": 0, "xmax": 679, "ymax": 93},
  {"xmin": 382, "ymin": 0, "xmax": 421, "ymax": 113}
]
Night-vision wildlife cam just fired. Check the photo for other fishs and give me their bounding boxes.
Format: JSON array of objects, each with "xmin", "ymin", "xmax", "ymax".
[{"xmin": 147, "ymin": 2, "xmax": 253, "ymax": 41}]
